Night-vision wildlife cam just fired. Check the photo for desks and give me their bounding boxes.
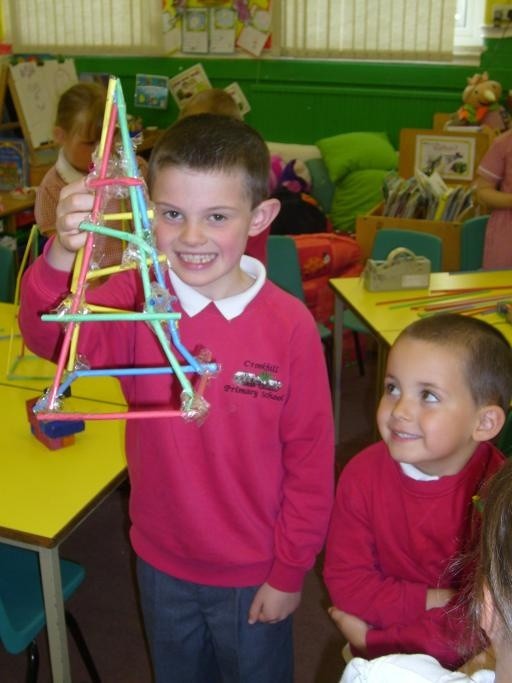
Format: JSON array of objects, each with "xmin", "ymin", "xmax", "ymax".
[
  {"xmin": 327, "ymin": 267, "xmax": 511, "ymax": 458},
  {"xmin": 0, "ymin": 300, "xmax": 126, "ymax": 683}
]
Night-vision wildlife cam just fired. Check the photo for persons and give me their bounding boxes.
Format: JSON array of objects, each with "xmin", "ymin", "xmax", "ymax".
[
  {"xmin": 33, "ymin": 84, "xmax": 154, "ymax": 270},
  {"xmin": 19, "ymin": 113, "xmax": 335, "ymax": 682},
  {"xmin": 323, "ymin": 312, "xmax": 512, "ymax": 669},
  {"xmin": 342, "ymin": 459, "xmax": 512, "ymax": 683},
  {"xmin": 476, "ymin": 126, "xmax": 512, "ymax": 270},
  {"xmin": 175, "ymin": 90, "xmax": 269, "ymax": 273}
]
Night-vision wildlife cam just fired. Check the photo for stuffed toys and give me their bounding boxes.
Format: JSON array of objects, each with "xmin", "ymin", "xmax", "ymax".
[
  {"xmin": 271, "ymin": 159, "xmax": 326, "ymax": 234},
  {"xmin": 448, "ymin": 70, "xmax": 509, "ymax": 134}
]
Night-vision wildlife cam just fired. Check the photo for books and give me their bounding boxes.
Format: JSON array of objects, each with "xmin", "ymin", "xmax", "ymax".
[{"xmin": 382, "ymin": 168, "xmax": 476, "ymax": 222}]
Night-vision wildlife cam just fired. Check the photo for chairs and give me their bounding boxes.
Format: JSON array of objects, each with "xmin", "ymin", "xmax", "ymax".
[
  {"xmin": 255, "ymin": 235, "xmax": 331, "ymax": 350},
  {"xmin": 461, "ymin": 217, "xmax": 490, "ymax": 269},
  {"xmin": 0, "ymin": 540, "xmax": 97, "ymax": 683},
  {"xmin": 331, "ymin": 231, "xmax": 440, "ymax": 378}
]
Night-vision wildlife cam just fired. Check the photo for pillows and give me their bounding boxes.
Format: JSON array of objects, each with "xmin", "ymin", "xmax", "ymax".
[{"xmin": 303, "ymin": 127, "xmax": 397, "ymax": 228}]
